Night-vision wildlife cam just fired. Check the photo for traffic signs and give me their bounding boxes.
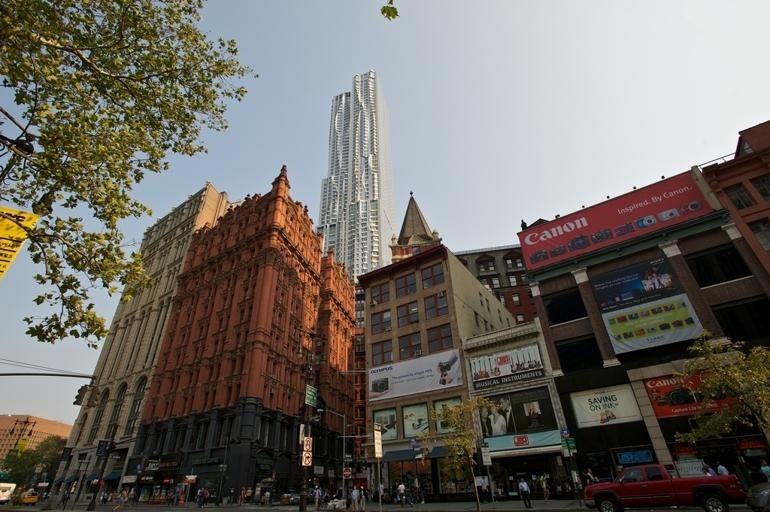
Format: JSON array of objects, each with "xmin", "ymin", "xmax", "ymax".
[{"xmin": 305, "ymin": 383, "xmax": 319, "ymax": 408}]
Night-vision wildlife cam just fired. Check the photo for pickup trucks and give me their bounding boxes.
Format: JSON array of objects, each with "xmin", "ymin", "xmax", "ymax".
[{"xmin": 580, "ymin": 461, "xmax": 745, "ymax": 511}]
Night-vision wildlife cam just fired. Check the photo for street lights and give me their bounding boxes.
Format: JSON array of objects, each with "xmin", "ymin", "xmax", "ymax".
[
  {"xmin": 217, "ymin": 464, "xmax": 228, "ymax": 506},
  {"xmin": 8, "ymin": 415, "xmax": 39, "ymax": 451},
  {"xmin": 317, "ymin": 408, "xmax": 350, "ymax": 502},
  {"xmin": 297, "ymin": 328, "xmax": 329, "ymax": 511}
]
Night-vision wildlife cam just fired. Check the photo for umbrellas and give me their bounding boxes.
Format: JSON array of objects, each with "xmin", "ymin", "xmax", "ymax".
[{"xmin": 261, "ymin": 477, "xmax": 278, "ymax": 483}]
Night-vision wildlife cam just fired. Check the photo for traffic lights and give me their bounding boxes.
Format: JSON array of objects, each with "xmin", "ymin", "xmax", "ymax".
[
  {"xmin": 104, "ymin": 441, "xmax": 111, "ymax": 455},
  {"xmin": 86, "ymin": 386, "xmax": 103, "ymax": 409},
  {"xmin": 72, "ymin": 385, "xmax": 88, "ymax": 406},
  {"xmin": 96, "ymin": 440, "xmax": 104, "ymax": 456}
]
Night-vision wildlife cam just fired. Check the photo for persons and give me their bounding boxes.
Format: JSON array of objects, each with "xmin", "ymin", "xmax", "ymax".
[
  {"xmin": 56, "ymin": 489, "xmax": 71, "ymax": 511},
  {"xmin": 498, "ymin": 395, "xmax": 514, "ymax": 436},
  {"xmin": 479, "ymin": 475, "xmax": 491, "ymax": 505},
  {"xmin": 488, "ymin": 403, "xmax": 507, "ymax": 435},
  {"xmin": 524, "ymin": 403, "xmax": 540, "ymax": 416},
  {"xmin": 745, "ymin": 469, "xmax": 768, "ymax": 484},
  {"xmin": 759, "ymin": 457, "xmax": 770, "ymax": 482},
  {"xmin": 479, "ymin": 403, "xmax": 493, "ymax": 436},
  {"xmin": 311, "ymin": 476, "xmax": 422, "ymax": 512},
  {"xmin": 641, "ymin": 269, "xmax": 673, "ymax": 298},
  {"xmin": 517, "ymin": 476, "xmax": 533, "ymax": 508},
  {"xmin": 715, "ymin": 461, "xmax": 729, "ymax": 475},
  {"xmin": 101, "ymin": 484, "xmax": 275, "ymax": 512},
  {"xmin": 533, "ymin": 467, "xmax": 600, "ymax": 511}
]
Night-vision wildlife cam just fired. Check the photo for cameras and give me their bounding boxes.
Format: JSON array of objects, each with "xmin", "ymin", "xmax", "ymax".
[
  {"xmin": 568, "ymin": 234, "xmax": 590, "ymax": 252},
  {"xmin": 529, "ymin": 248, "xmax": 549, "ymax": 264},
  {"xmin": 615, "ymin": 221, "xmax": 635, "ymax": 238},
  {"xmin": 680, "ymin": 199, "xmax": 704, "ymax": 216},
  {"xmin": 658, "ymin": 207, "xmax": 680, "ymax": 222},
  {"xmin": 550, "ymin": 244, "xmax": 567, "ymax": 258},
  {"xmin": 372, "ymin": 378, "xmax": 388, "ymax": 393},
  {"xmin": 637, "ymin": 215, "xmax": 657, "ymax": 229},
  {"xmin": 608, "ymin": 302, "xmax": 695, "ymax": 340},
  {"xmin": 665, "ymin": 386, "xmax": 694, "ymax": 405},
  {"xmin": 591, "ymin": 228, "xmax": 614, "ymax": 245}
]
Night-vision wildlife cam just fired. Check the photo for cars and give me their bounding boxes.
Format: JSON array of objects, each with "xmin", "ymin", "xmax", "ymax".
[
  {"xmin": 0, "ymin": 481, "xmax": 43, "ymax": 507},
  {"xmin": 279, "ymin": 486, "xmax": 358, "ymax": 506},
  {"xmin": 744, "ymin": 482, "xmax": 770, "ymax": 512}
]
